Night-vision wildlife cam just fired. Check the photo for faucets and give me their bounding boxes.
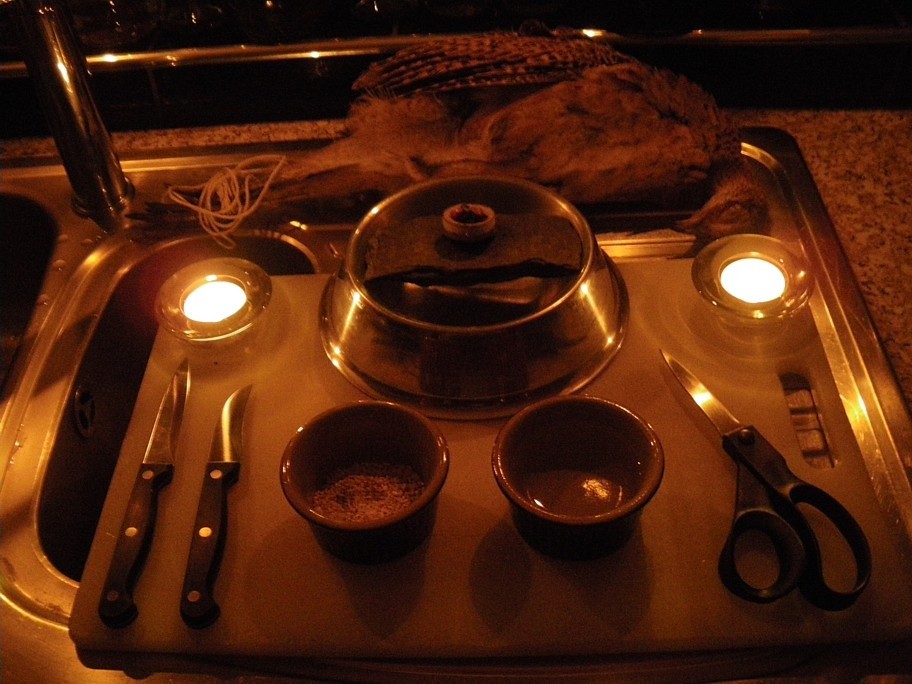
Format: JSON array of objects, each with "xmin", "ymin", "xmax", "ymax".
[{"xmin": 10, "ymin": 8, "xmax": 137, "ymax": 218}]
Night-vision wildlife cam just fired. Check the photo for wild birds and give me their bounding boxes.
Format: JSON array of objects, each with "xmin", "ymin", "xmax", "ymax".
[{"xmin": 126, "ymin": 34, "xmax": 770, "ymax": 254}]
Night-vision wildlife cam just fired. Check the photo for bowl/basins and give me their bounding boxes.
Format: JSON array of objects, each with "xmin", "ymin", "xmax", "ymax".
[
  {"xmin": 331, "ymin": 175, "xmax": 615, "ymax": 399},
  {"xmin": 491, "ymin": 394, "xmax": 664, "ymax": 523},
  {"xmin": 280, "ymin": 402, "xmax": 449, "ymax": 536}
]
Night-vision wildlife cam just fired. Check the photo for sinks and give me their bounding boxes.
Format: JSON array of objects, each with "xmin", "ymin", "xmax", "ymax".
[
  {"xmin": 35, "ymin": 233, "xmax": 316, "ymax": 589},
  {"xmin": 1, "ymin": 189, "xmax": 61, "ymax": 425}
]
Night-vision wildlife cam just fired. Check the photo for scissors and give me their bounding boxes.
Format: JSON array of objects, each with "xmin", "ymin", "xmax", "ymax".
[{"xmin": 658, "ymin": 346, "xmax": 871, "ymax": 615}]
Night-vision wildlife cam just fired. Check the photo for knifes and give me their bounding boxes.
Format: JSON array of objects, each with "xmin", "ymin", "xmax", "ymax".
[
  {"xmin": 178, "ymin": 382, "xmax": 254, "ymax": 623},
  {"xmin": 98, "ymin": 350, "xmax": 191, "ymax": 624}
]
